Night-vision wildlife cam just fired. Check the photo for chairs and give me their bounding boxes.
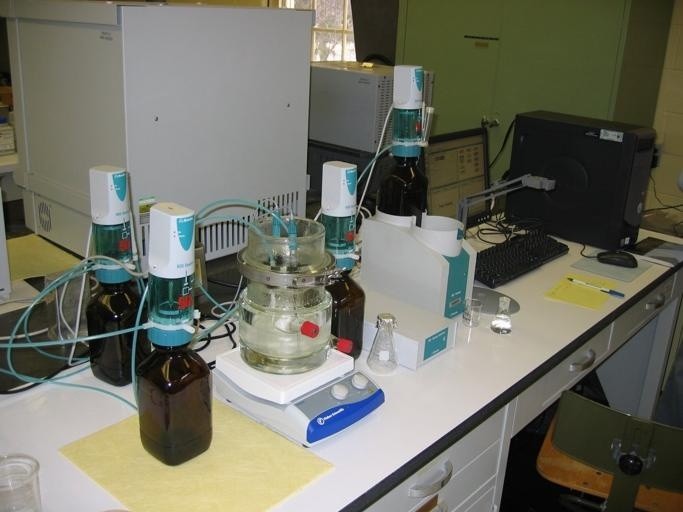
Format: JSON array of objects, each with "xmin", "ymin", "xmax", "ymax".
[{"xmin": 535, "ymin": 389, "xmax": 683, "ymax": 512}]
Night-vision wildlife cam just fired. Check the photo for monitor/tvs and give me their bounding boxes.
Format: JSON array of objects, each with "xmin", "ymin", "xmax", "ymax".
[{"xmin": 421, "ymin": 127, "xmax": 491, "ymax": 230}]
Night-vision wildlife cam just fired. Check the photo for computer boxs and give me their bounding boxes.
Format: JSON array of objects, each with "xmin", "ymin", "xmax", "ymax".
[{"xmin": 504, "ymin": 108, "xmax": 656, "ymax": 251}]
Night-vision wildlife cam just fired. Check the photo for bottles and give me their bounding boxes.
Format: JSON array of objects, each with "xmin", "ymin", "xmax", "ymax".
[
  {"xmin": 132, "ymin": 327, "xmax": 214, "ymax": 466},
  {"xmin": 365, "ymin": 312, "xmax": 399, "ymax": 375},
  {"xmin": 1, "ymin": 455, "xmax": 43, "ymax": 511},
  {"xmin": 330, "ymin": 253, "xmax": 368, "ymax": 363},
  {"xmin": 86, "ymin": 266, "xmax": 149, "ymax": 387},
  {"xmin": 377, "ymin": 140, "xmax": 431, "ymax": 228},
  {"xmin": 490, "ymin": 297, "xmax": 513, "ymax": 335}
]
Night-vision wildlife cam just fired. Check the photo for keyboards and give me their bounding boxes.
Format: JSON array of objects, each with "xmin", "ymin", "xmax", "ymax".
[{"xmin": 474, "ymin": 228, "xmax": 569, "ymax": 288}]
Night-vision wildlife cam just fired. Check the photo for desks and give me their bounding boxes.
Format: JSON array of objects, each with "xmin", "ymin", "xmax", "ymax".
[{"xmin": 0, "ymin": 213, "xmax": 683, "ymax": 512}]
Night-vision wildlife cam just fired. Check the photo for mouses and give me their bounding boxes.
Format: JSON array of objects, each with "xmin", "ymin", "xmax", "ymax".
[{"xmin": 597, "ymin": 249, "xmax": 639, "ymax": 268}]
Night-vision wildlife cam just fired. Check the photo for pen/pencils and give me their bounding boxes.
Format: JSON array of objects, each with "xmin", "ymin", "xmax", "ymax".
[{"xmin": 566, "ymin": 277, "xmax": 625, "ymax": 299}]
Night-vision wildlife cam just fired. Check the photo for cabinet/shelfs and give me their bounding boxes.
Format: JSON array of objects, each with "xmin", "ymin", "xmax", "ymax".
[
  {"xmin": 464, "ymin": 486, "xmax": 494, "ymax": 512},
  {"xmin": 363, "ymin": 404, "xmax": 506, "ymax": 512},
  {"xmin": 511, "ymin": 323, "xmax": 612, "ymax": 437},
  {"xmin": 609, "ymin": 273, "xmax": 675, "ymax": 351},
  {"xmin": 671, "ymin": 267, "xmax": 683, "ymax": 299},
  {"xmin": 410, "ymin": 438, "xmax": 501, "ymax": 512}
]
